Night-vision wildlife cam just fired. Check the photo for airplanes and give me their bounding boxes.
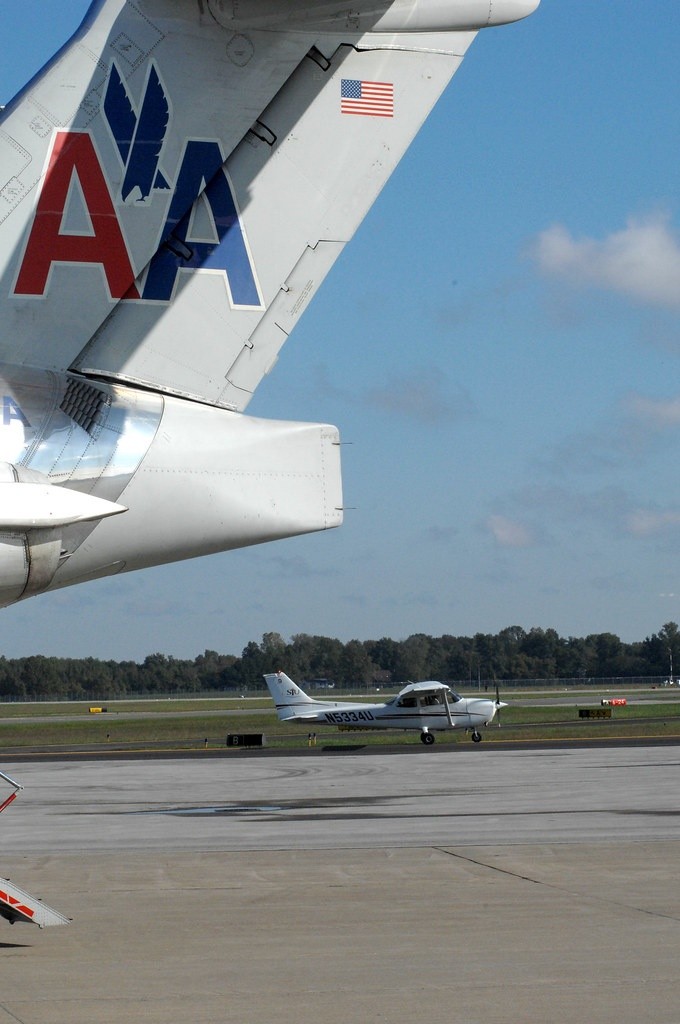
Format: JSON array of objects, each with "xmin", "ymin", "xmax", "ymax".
[
  {"xmin": 263, "ymin": 671, "xmax": 507, "ymax": 745},
  {"xmin": 0, "ymin": 0, "xmax": 541, "ymax": 931}
]
